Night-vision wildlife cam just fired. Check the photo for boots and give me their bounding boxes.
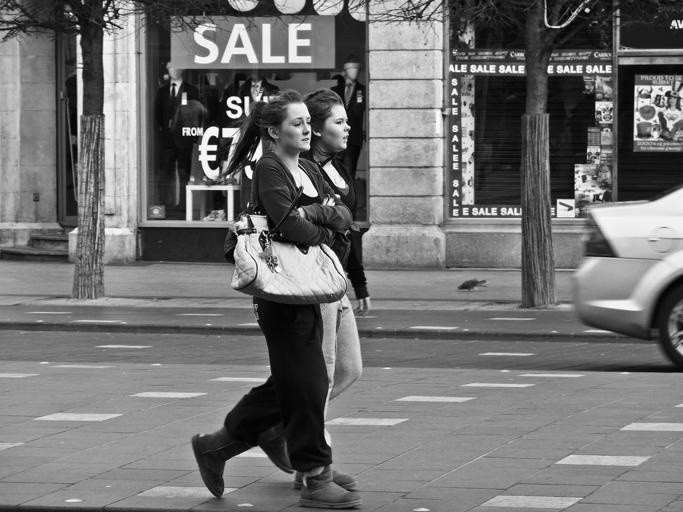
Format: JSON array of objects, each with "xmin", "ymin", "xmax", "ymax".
[
  {"xmin": 192, "ymin": 426, "xmax": 252, "ymax": 498},
  {"xmin": 299, "ymin": 464, "xmax": 363, "ymax": 509}
]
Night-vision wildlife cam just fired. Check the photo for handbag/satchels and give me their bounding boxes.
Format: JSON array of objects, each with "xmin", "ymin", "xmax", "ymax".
[{"xmin": 229, "ymin": 169, "xmax": 348, "ymax": 306}]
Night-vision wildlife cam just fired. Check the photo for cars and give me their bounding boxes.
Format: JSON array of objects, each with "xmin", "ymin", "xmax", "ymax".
[{"xmin": 569, "ymin": 182, "xmax": 683, "ymax": 370}]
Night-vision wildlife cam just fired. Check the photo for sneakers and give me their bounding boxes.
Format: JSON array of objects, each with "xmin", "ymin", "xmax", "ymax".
[
  {"xmin": 294, "ymin": 466, "xmax": 358, "ymax": 490},
  {"xmin": 259, "ymin": 436, "xmax": 295, "ymax": 474}
]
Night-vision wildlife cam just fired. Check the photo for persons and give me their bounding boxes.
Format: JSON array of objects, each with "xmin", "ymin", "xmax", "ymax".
[
  {"xmin": 659, "ymin": 95, "xmax": 682, "ymax": 143},
  {"xmin": 153, "ymin": 61, "xmax": 202, "ymax": 213},
  {"xmin": 200, "ymin": 72, "xmax": 238, "ymax": 222},
  {"xmin": 230, "ymin": 69, "xmax": 283, "ymax": 206},
  {"xmin": 188, "ymin": 90, "xmax": 365, "ymax": 510},
  {"xmin": 245, "ymin": 84, "xmax": 371, "ymax": 497},
  {"xmin": 330, "ymin": 53, "xmax": 366, "ymax": 186}
]
